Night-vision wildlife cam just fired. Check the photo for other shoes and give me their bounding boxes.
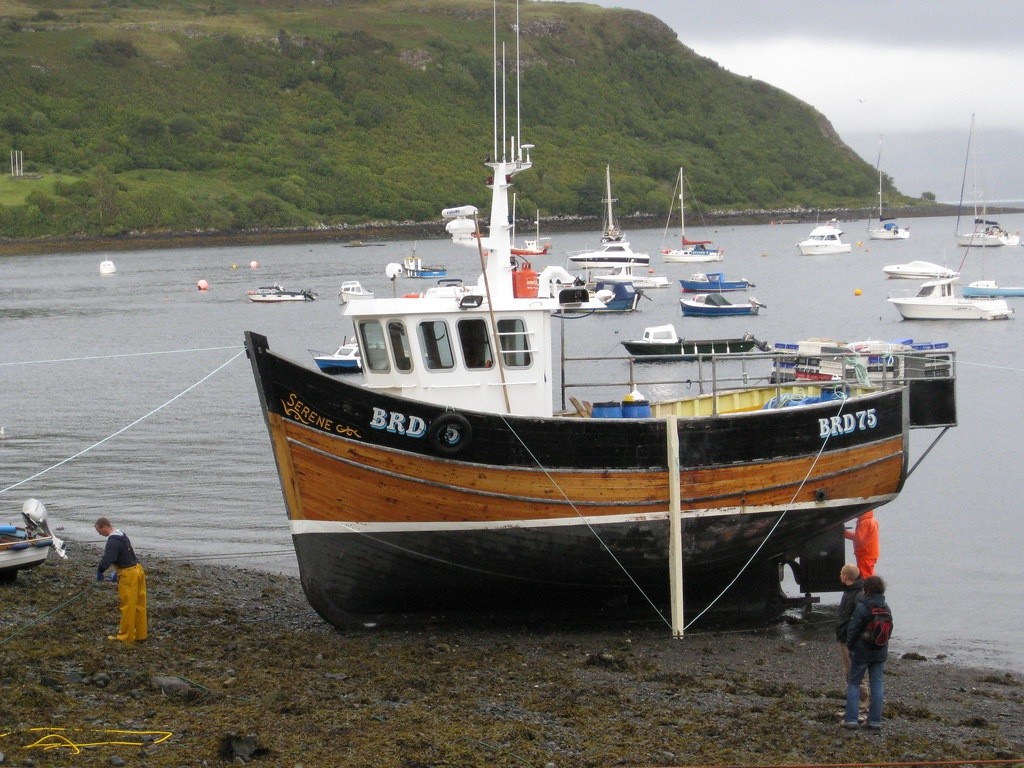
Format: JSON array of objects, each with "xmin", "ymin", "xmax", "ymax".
[
  {"xmin": 866, "ymin": 720, "xmax": 882, "ymax": 729},
  {"xmin": 107, "ymin": 635, "xmax": 117, "ymax": 640},
  {"xmin": 840, "ymin": 719, "xmax": 858, "ymax": 730},
  {"xmin": 858, "ymin": 712, "xmax": 868, "ymax": 722}
]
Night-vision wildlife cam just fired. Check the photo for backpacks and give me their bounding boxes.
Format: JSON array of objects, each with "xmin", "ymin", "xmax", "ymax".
[{"xmin": 859, "ymin": 599, "xmax": 893, "ymax": 649}]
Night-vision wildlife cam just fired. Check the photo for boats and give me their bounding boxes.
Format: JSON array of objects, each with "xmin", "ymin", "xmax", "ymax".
[
  {"xmin": 593, "ymin": 264, "xmax": 672, "ymax": 288},
  {"xmin": 306, "ymin": 334, "xmax": 411, "ymax": 374},
  {"xmin": 620, "ymin": 325, "xmax": 774, "ymax": 363},
  {"xmin": 241, "ymin": 0, "xmax": 958, "ymax": 631},
  {"xmin": 247, "ymin": 282, "xmax": 319, "ymax": 302},
  {"xmin": 339, "ymin": 281, "xmax": 376, "ymax": 305},
  {"xmin": 677, "ymin": 271, "xmax": 755, "ymax": 293},
  {"xmin": 795, "ymin": 208, "xmax": 852, "ymax": 256},
  {"xmin": 882, "ymin": 261, "xmax": 961, "ymax": 280},
  {"xmin": 555, "ymin": 280, "xmax": 655, "ymax": 314},
  {"xmin": 887, "ymin": 277, "xmax": 1013, "ymax": 322},
  {"xmin": 99, "ymin": 254, "xmax": 116, "ymax": 274},
  {"xmin": 402, "ymin": 248, "xmax": 447, "ymax": 279},
  {"xmin": 0, "ymin": 498, "xmax": 69, "ymax": 583},
  {"xmin": 679, "ymin": 292, "xmax": 768, "ymax": 316}
]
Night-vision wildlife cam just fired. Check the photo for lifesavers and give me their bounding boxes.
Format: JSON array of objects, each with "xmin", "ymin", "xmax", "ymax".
[{"xmin": 426, "ymin": 413, "xmax": 472, "ymax": 454}]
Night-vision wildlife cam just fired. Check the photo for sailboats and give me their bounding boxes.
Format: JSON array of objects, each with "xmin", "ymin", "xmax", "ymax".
[
  {"xmin": 961, "ymin": 202, "xmax": 1024, "ymax": 297},
  {"xmin": 567, "ymin": 162, "xmax": 651, "ymax": 267},
  {"xmin": 483, "ymin": 191, "xmax": 551, "ymax": 257},
  {"xmin": 866, "ymin": 133, "xmax": 911, "ymax": 240},
  {"xmin": 659, "ymin": 166, "xmax": 725, "ymax": 263},
  {"xmin": 954, "ymin": 111, "xmax": 1020, "ymax": 248}
]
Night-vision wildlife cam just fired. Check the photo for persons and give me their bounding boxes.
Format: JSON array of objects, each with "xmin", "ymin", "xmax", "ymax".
[
  {"xmin": 846, "ymin": 510, "xmax": 879, "ymax": 581},
  {"xmin": 837, "ymin": 567, "xmax": 868, "ymax": 726},
  {"xmin": 840, "ymin": 575, "xmax": 892, "ymax": 731},
  {"xmin": 95, "ymin": 517, "xmax": 148, "ymax": 644}
]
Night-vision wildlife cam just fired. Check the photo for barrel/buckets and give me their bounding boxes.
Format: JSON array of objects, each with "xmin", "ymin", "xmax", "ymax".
[
  {"xmin": 592, "ymin": 402, "xmax": 623, "ymax": 418},
  {"xmin": 622, "ymin": 400, "xmax": 651, "ymax": 418},
  {"xmin": 512, "ymin": 262, "xmax": 538, "ymax": 298},
  {"xmin": 821, "ymin": 385, "xmax": 850, "ymax": 403}
]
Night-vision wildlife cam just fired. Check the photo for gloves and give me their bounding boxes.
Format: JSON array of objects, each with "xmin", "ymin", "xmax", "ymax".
[
  {"xmin": 96, "ymin": 572, "xmax": 104, "ymax": 584},
  {"xmin": 110, "ymin": 571, "xmax": 117, "ymax": 582}
]
